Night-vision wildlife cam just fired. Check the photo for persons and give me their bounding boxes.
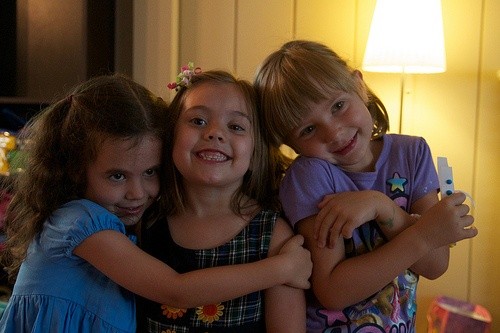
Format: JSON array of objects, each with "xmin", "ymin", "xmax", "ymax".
[{"xmin": 1, "ymin": 39, "xmax": 479, "ymax": 332}]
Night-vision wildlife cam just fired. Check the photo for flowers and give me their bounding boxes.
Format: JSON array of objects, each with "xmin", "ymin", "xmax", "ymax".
[{"xmin": 167, "ymin": 61, "xmax": 202, "ymax": 92}]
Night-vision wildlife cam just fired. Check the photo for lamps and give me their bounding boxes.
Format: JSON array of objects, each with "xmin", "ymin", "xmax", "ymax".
[{"xmin": 362, "ymin": 0, "xmax": 446, "ymax": 135}]
